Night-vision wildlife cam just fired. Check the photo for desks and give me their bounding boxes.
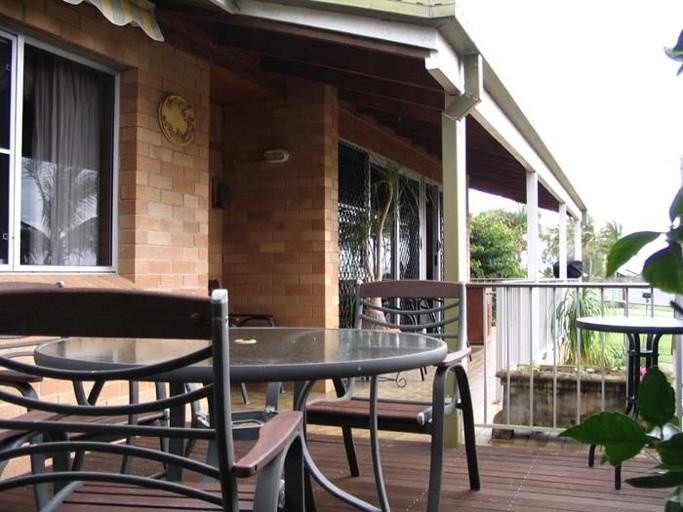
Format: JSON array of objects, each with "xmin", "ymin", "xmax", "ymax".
[
  {"xmin": 32, "ymin": 326, "xmax": 446, "ymax": 512},
  {"xmin": 577, "ymin": 314, "xmax": 682, "ymax": 492}
]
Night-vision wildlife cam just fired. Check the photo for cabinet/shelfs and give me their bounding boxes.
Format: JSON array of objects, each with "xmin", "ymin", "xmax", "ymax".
[{"xmin": 467, "ymin": 281, "xmax": 493, "ymax": 346}]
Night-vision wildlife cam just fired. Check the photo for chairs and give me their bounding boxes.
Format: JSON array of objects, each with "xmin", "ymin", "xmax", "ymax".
[
  {"xmin": 0, "ymin": 280, "xmax": 197, "ymax": 512},
  {"xmin": 208, "ymin": 278, "xmax": 284, "ymax": 403},
  {"xmin": 304, "ymin": 279, "xmax": 481, "ymax": 510},
  {"xmin": 0, "ymin": 280, "xmax": 305, "ymax": 512}
]
